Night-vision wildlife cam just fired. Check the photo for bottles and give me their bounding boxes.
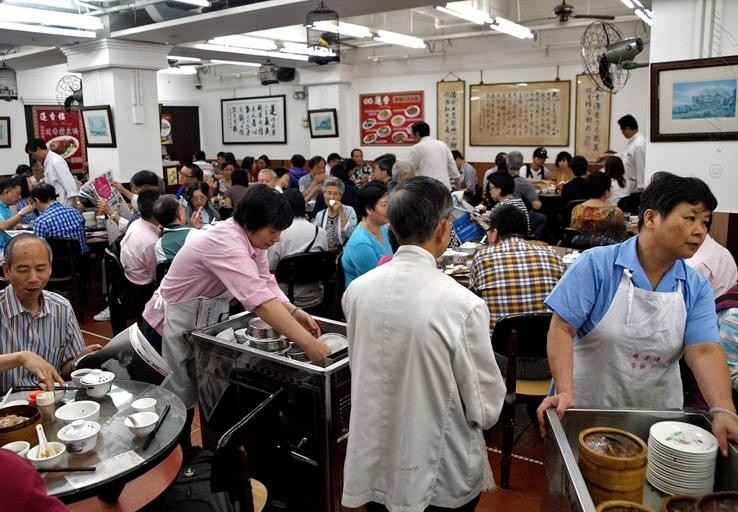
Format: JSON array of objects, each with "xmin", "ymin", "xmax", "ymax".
[{"xmin": 28, "ymin": 389, "xmax": 57, "ymax": 426}]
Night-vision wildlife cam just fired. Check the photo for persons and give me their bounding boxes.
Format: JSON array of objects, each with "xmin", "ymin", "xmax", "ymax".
[
  {"xmin": 400, "ymin": 121, "xmax": 461, "ymax": 192},
  {"xmin": 94, "ymin": 149, "xmax": 738, "ymax": 431},
  {"xmin": 339, "ymin": 177, "xmax": 506, "ymax": 511},
  {"xmin": 26, "ymin": 138, "xmax": 87, "ymax": 214},
  {"xmin": 618, "ymin": 114, "xmax": 646, "ymax": 193},
  {"xmin": 1, "ymin": 162, "xmax": 103, "ymax": 512},
  {"xmin": 537, "ymin": 172, "xmax": 738, "ymax": 457}
]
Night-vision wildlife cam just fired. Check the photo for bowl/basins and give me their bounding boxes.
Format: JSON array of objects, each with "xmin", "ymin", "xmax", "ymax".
[
  {"xmin": 54, "ymin": 400, "xmax": 101, "ymax": 428},
  {"xmin": 53, "ymin": 382, "xmax": 68, "ymax": 404},
  {"xmin": 70, "ymin": 367, "xmax": 91, "ymax": 390},
  {"xmin": 234, "ymin": 328, "xmax": 249, "ymax": 346},
  {"xmin": 1, "ymin": 440, "xmax": 30, "ymax": 459},
  {"xmin": 242, "ymin": 315, "xmax": 313, "ymax": 365},
  {"xmin": 25, "ymin": 442, "xmax": 67, "ymax": 468},
  {"xmin": 124, "ymin": 411, "xmax": 160, "ymax": 439}
]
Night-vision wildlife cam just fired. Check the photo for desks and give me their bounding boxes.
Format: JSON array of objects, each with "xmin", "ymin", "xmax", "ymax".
[{"xmin": 0, "ymin": 378, "xmax": 186, "ymax": 512}]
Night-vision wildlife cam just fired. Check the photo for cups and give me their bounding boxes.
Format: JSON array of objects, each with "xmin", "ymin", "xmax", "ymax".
[
  {"xmin": 82, "ymin": 369, "xmax": 116, "ymax": 399},
  {"xmin": 131, "ymin": 397, "xmax": 157, "ymax": 413},
  {"xmin": 57, "ymin": 420, "xmax": 101, "ymax": 453},
  {"xmin": 541, "ymin": 186, "xmax": 556, "ymax": 194},
  {"xmin": 84, "ymin": 220, "xmax": 96, "ymax": 226},
  {"xmin": 82, "ymin": 212, "xmax": 95, "ymax": 220},
  {"xmin": 436, "ymin": 242, "xmax": 484, "ymax": 275}
]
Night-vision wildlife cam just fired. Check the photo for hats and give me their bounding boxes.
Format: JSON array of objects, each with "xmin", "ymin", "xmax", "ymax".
[{"xmin": 533, "ymin": 147, "xmax": 549, "ymax": 159}]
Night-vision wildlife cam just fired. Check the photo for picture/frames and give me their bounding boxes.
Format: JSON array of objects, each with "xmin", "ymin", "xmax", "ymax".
[
  {"xmin": 220, "ymin": 93, "xmax": 287, "ymax": 145},
  {"xmin": 0, "ymin": 116, "xmax": 13, "ymax": 149},
  {"xmin": 434, "ymin": 78, "xmax": 466, "ymax": 161},
  {"xmin": 573, "ymin": 72, "xmax": 613, "ymax": 163},
  {"xmin": 648, "ymin": 53, "xmax": 737, "ymax": 143},
  {"xmin": 467, "ymin": 79, "xmax": 571, "ymax": 147},
  {"xmin": 306, "ymin": 107, "xmax": 339, "ymax": 138},
  {"xmin": 79, "ymin": 102, "xmax": 117, "ymax": 151}
]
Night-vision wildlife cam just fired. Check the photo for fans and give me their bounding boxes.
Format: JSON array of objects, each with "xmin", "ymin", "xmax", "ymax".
[
  {"xmin": 53, "ymin": 73, "xmax": 84, "ymax": 122},
  {"xmin": 513, "ymin": 0, "xmax": 619, "ymax": 29},
  {"xmin": 575, "ymin": 18, "xmax": 649, "ymax": 93}
]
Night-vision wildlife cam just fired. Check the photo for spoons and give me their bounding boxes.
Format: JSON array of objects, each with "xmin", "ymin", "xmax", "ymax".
[{"xmin": 36, "ymin": 424, "xmax": 56, "ymax": 460}]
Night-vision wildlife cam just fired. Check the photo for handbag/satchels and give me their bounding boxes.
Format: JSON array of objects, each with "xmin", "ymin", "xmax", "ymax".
[{"xmin": 156, "ymin": 449, "xmax": 240, "ymax": 512}]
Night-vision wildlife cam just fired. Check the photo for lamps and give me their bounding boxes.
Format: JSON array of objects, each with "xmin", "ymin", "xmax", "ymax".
[{"xmin": 37, "ymin": 0, "xmax": 538, "ymax": 64}]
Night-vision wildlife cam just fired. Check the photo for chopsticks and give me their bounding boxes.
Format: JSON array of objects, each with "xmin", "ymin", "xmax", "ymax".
[
  {"xmin": 12, "ymin": 385, "xmax": 94, "ymax": 392},
  {"xmin": 141, "ymin": 403, "xmax": 171, "ymax": 451},
  {"xmin": 34, "ymin": 468, "xmax": 97, "ymax": 472}
]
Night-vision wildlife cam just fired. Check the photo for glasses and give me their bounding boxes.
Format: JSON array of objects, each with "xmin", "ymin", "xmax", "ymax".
[{"xmin": 178, "ymin": 170, "xmax": 195, "ymax": 179}]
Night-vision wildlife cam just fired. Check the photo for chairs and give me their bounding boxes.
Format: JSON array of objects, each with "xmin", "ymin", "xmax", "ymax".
[{"xmin": 3, "ymin": 178, "xmax": 632, "ymax": 489}]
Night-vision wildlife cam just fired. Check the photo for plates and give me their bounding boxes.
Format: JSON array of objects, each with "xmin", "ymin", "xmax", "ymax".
[
  {"xmin": 160, "ymin": 118, "xmax": 172, "ymax": 137},
  {"xmin": 315, "ymin": 332, "xmax": 350, "ymax": 355},
  {"xmin": 4, "ymin": 399, "xmax": 30, "ymax": 408},
  {"xmin": 364, "ymin": 107, "xmax": 421, "ymax": 145},
  {"xmin": 644, "ymin": 420, "xmax": 719, "ymax": 497},
  {"xmin": 45, "ymin": 136, "xmax": 80, "ymax": 160}
]
